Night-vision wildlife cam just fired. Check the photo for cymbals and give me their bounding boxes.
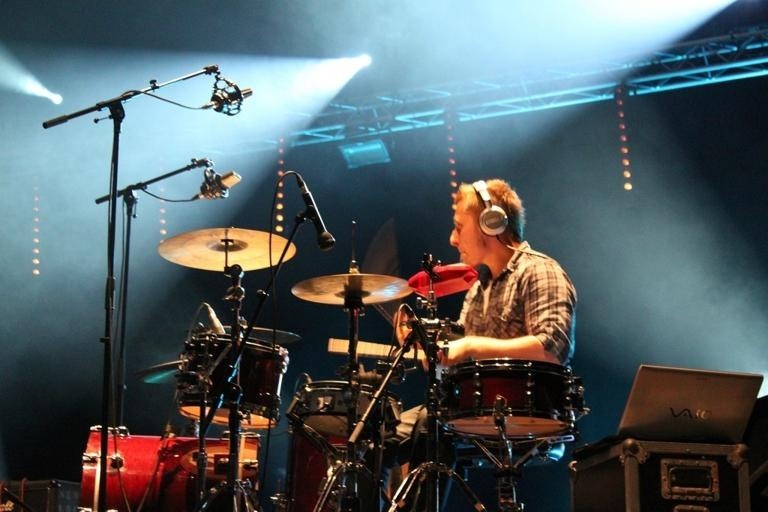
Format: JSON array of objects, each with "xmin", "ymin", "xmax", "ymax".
[
  {"xmin": 221, "ymin": 324, "xmax": 303, "ymax": 348},
  {"xmin": 156, "ymin": 226, "xmax": 296, "ymax": 273},
  {"xmin": 289, "ymin": 273, "xmax": 412, "ymax": 307}
]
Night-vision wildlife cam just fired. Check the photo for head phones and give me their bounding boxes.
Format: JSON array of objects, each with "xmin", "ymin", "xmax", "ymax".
[{"xmin": 473, "ymin": 180, "xmax": 509, "ymax": 237}]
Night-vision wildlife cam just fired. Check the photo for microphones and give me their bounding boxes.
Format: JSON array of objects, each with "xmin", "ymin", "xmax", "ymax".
[
  {"xmin": 202, "ymin": 87, "xmax": 253, "ymax": 108},
  {"xmin": 295, "ymin": 173, "xmax": 337, "ymax": 252},
  {"xmin": 404, "ymin": 302, "xmax": 440, "ymax": 365},
  {"xmin": 192, "ymin": 169, "xmax": 243, "ymax": 201},
  {"xmin": 204, "ymin": 302, "xmax": 226, "ymax": 335}
]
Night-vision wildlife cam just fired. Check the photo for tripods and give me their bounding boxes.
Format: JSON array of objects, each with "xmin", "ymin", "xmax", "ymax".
[
  {"xmin": 312, "ymin": 300, "xmax": 393, "ymax": 512},
  {"xmin": 196, "ymin": 288, "xmax": 264, "ymax": 512},
  {"xmin": 388, "ymin": 369, "xmax": 487, "ymax": 512}
]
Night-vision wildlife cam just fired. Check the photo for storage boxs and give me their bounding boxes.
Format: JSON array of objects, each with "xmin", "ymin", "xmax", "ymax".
[
  {"xmin": 0, "ymin": 478, "xmax": 81, "ymax": 511},
  {"xmin": 568, "ymin": 437, "xmax": 751, "ymax": 511}
]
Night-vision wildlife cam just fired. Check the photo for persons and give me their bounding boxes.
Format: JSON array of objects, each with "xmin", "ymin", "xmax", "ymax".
[{"xmin": 357, "ymin": 179, "xmax": 577, "ymax": 512}]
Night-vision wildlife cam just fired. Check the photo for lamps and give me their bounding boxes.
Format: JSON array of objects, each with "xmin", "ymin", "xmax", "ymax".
[{"xmin": 336, "ymin": 136, "xmax": 393, "ymax": 170}]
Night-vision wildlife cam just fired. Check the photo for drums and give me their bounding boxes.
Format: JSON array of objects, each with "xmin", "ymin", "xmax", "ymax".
[
  {"xmin": 294, "ymin": 379, "xmax": 400, "ymax": 440},
  {"xmin": 80, "ymin": 427, "xmax": 260, "ymax": 512},
  {"xmin": 175, "ymin": 336, "xmax": 290, "ymax": 430},
  {"xmin": 282, "ymin": 433, "xmax": 381, "ymax": 512},
  {"xmin": 397, "ymin": 367, "xmax": 437, "ymax": 440},
  {"xmin": 430, "ymin": 357, "xmax": 576, "ymax": 446}
]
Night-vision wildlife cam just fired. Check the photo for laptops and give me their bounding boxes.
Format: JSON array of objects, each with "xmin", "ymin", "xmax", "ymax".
[{"xmin": 571, "ymin": 364, "xmax": 764, "ymax": 460}]
